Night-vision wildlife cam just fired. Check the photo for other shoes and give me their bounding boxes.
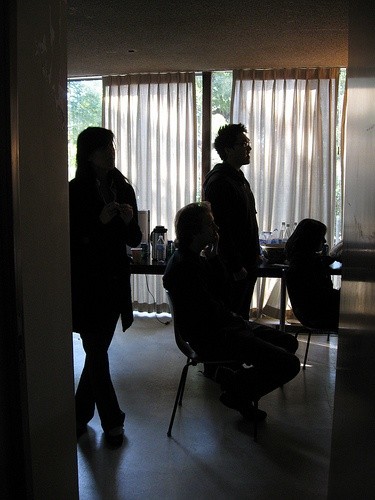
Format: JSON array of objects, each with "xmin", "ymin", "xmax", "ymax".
[
  {"xmin": 222, "ymin": 389, "xmax": 268, "ymax": 419},
  {"xmin": 214, "ymin": 366, "xmax": 236, "ymax": 387},
  {"xmin": 75, "ymin": 425, "xmax": 87, "ymax": 441},
  {"xmin": 105, "ymin": 433, "xmax": 122, "ymax": 450}
]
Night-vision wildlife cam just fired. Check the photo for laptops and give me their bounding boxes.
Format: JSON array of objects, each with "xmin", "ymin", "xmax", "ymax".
[{"xmin": 126, "ymin": 209, "xmax": 150, "ymax": 255}]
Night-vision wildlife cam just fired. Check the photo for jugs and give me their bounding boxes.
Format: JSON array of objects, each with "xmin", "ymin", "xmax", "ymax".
[{"xmin": 150, "ymin": 226, "xmax": 168, "ymax": 261}]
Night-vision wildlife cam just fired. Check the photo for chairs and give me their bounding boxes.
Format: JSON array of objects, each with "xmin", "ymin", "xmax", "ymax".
[
  {"xmin": 166, "ymin": 290, "xmax": 262, "ymax": 442},
  {"xmin": 281, "ymin": 271, "xmax": 340, "ymax": 369}
]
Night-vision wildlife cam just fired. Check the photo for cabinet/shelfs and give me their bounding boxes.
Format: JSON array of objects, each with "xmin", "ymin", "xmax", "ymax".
[{"xmin": 324, "ymin": 280, "xmax": 375, "ymax": 500}]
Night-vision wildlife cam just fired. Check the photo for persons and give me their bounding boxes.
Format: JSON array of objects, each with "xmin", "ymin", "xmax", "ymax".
[
  {"xmin": 69, "ymin": 128, "xmax": 143, "ymax": 449},
  {"xmin": 163, "ymin": 203, "xmax": 300, "ymax": 420},
  {"xmin": 284, "ymin": 219, "xmax": 340, "ymax": 331},
  {"xmin": 203, "ymin": 124, "xmax": 264, "ymax": 381}
]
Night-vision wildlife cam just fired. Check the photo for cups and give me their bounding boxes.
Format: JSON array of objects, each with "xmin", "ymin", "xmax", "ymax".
[
  {"xmin": 131, "ymin": 248, "xmax": 142, "ymax": 263},
  {"xmin": 261, "ymin": 231, "xmax": 273, "ymax": 245}
]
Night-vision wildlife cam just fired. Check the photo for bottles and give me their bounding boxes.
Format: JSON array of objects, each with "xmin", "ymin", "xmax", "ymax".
[
  {"xmin": 269, "ymin": 222, "xmax": 299, "ymax": 246},
  {"xmin": 157, "ymin": 236, "xmax": 166, "ymax": 262}
]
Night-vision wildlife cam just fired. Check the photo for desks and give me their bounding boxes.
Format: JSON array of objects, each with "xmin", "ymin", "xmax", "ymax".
[{"xmin": 128, "ymin": 262, "xmax": 286, "ymax": 325}]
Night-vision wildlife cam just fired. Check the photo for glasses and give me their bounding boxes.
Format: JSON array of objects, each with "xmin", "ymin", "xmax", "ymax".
[{"xmin": 233, "ymin": 138, "xmax": 250, "ymax": 148}]
